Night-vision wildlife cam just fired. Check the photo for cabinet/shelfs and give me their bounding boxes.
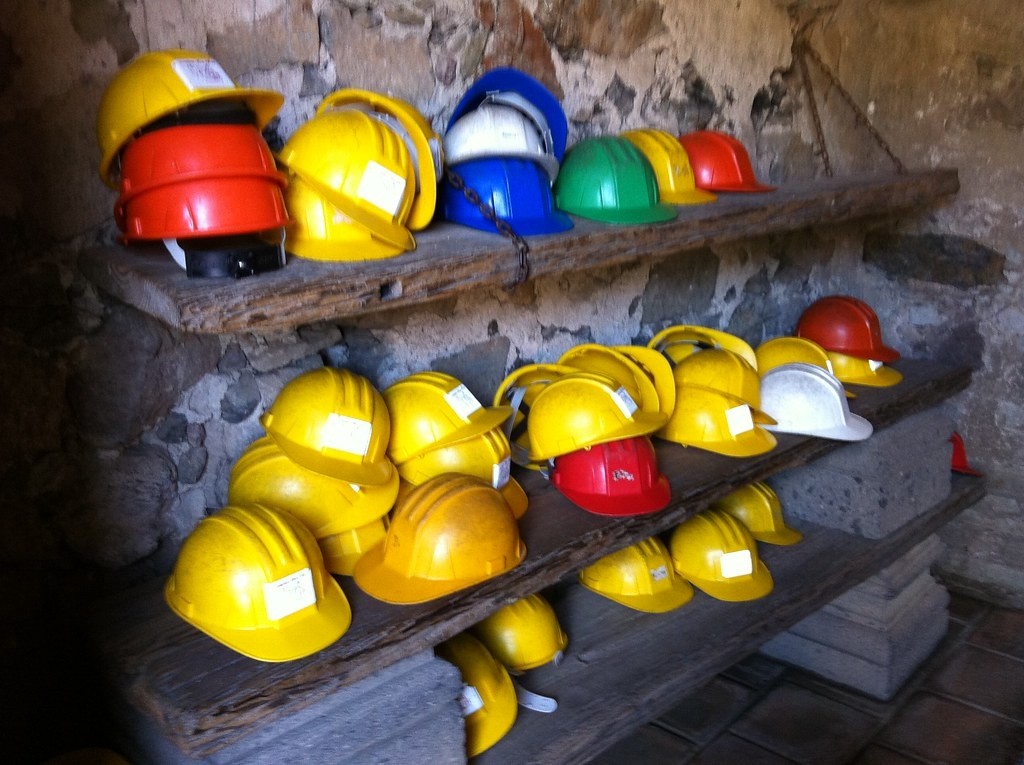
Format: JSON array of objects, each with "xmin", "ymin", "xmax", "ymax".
[{"xmin": 89, "ymin": 166, "xmax": 987, "ymax": 765}]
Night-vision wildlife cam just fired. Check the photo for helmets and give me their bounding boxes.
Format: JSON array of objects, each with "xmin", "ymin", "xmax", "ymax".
[
  {"xmin": 678, "ymin": 129, "xmax": 782, "ymax": 195},
  {"xmin": 619, "ymin": 129, "xmax": 718, "ymax": 206},
  {"xmin": 277, "ymin": 86, "xmax": 446, "ymax": 263},
  {"xmin": 948, "ymin": 430, "xmax": 985, "ymax": 478},
  {"xmin": 163, "ymin": 293, "xmax": 907, "ymax": 757},
  {"xmin": 95, "ymin": 44, "xmax": 295, "ymax": 277},
  {"xmin": 554, "ymin": 132, "xmax": 679, "ymax": 227},
  {"xmin": 440, "ymin": 63, "xmax": 574, "ymax": 235}
]
